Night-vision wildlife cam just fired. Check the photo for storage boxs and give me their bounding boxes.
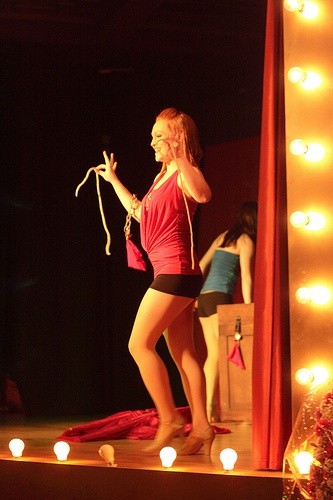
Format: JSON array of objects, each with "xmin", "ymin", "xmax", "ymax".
[{"xmin": 216, "ymin": 303, "xmax": 254, "ymax": 422}]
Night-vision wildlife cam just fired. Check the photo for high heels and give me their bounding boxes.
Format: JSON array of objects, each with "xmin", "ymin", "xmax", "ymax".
[
  {"xmin": 173, "ymin": 427, "xmax": 215, "ymax": 457},
  {"xmin": 205, "ymin": 404, "xmax": 220, "ymax": 424},
  {"xmin": 141, "ymin": 416, "xmax": 186, "ymax": 453}
]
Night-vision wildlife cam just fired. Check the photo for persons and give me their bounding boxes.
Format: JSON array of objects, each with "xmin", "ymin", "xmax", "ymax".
[
  {"xmin": 94, "ymin": 109, "xmax": 215, "ymax": 456},
  {"xmin": 194, "ymin": 201, "xmax": 256, "ymax": 424}
]
